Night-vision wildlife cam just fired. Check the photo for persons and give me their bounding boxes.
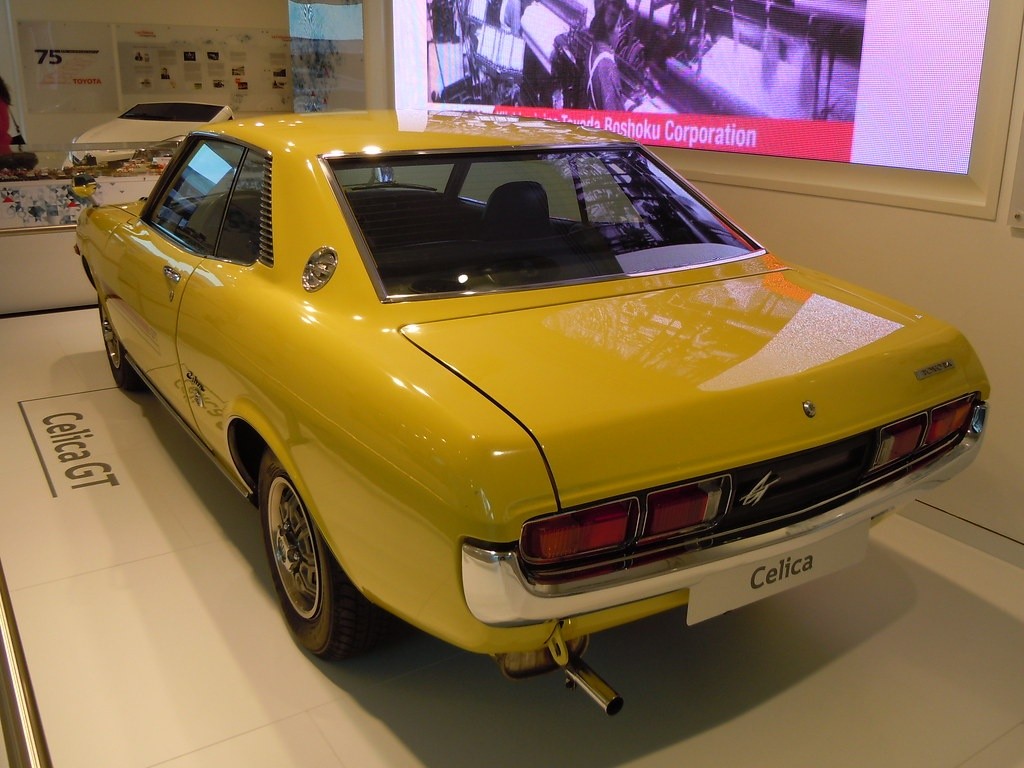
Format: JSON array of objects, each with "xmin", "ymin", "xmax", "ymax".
[
  {"xmin": 0, "ymin": 75, "xmax": 14, "ymax": 183},
  {"xmin": 549, "ymin": 0, "xmax": 637, "ymax": 112}
]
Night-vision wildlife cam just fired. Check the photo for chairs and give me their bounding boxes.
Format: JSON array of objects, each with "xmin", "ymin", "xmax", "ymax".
[{"xmin": 477, "ymin": 182, "xmax": 552, "ymax": 241}]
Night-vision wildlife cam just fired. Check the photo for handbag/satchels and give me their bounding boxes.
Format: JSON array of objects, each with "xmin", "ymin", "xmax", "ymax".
[{"xmin": 10, "ymin": 133, "xmax": 26, "ymax": 145}]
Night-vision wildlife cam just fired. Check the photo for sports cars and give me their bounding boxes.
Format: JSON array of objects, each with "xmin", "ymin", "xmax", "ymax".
[
  {"xmin": 73, "ymin": 111, "xmax": 991, "ymax": 717},
  {"xmin": 61, "ymin": 98, "xmax": 234, "ymax": 172}
]
